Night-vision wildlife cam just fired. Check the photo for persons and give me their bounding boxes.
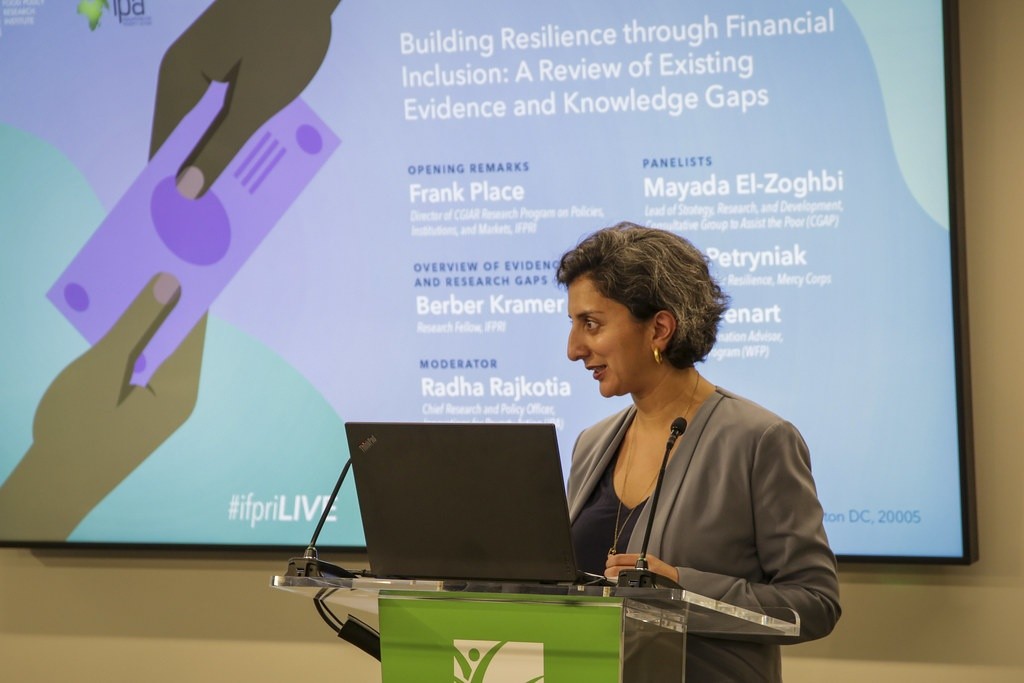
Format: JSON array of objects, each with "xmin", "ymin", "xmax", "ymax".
[{"xmin": 554, "ymin": 221, "xmax": 843, "ymax": 682}]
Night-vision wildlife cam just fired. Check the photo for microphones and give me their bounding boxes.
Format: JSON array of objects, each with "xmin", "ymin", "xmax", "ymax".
[{"xmin": 618, "ymin": 417, "xmax": 689, "ymax": 591}]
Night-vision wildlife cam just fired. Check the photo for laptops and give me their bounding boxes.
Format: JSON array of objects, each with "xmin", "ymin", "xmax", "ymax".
[{"xmin": 345, "ymin": 422, "xmax": 579, "ymax": 582}]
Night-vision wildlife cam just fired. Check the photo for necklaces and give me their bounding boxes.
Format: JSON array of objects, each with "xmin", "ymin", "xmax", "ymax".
[{"xmin": 608, "ymin": 371, "xmax": 699, "ymax": 555}]
